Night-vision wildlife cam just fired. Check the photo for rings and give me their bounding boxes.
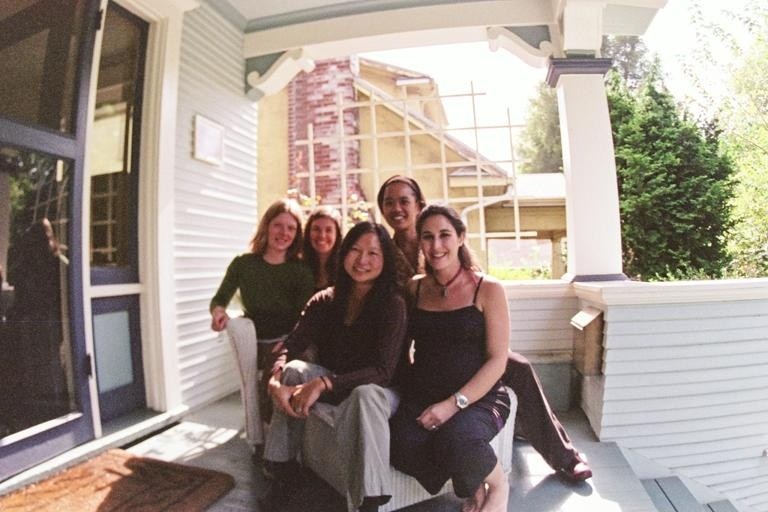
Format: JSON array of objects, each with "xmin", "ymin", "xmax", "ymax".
[
  {"xmin": 294, "ymin": 407, "xmax": 302, "ymax": 412},
  {"xmin": 432, "ymin": 425, "xmax": 437, "ymax": 428}
]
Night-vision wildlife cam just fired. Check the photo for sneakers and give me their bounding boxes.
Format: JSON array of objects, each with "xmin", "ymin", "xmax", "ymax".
[{"xmin": 563, "ymin": 456, "xmax": 593, "ymax": 480}]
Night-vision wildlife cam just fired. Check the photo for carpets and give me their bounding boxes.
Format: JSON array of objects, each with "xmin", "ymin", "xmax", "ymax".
[{"xmin": 0, "ymin": 448, "xmax": 238, "ymax": 512}]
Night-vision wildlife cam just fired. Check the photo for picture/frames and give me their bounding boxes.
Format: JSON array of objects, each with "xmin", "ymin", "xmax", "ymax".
[{"xmin": 192, "ymin": 113, "xmax": 226, "ymax": 166}]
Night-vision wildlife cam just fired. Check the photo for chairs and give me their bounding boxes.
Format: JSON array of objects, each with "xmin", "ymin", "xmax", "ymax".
[
  {"xmin": 265, "ymin": 375, "xmax": 519, "ymax": 512},
  {"xmin": 225, "ymin": 317, "xmax": 265, "ymax": 454}
]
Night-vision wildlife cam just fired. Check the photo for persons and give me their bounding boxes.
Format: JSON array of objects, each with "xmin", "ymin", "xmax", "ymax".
[
  {"xmin": 4, "ymin": 217, "xmax": 68, "ymax": 318},
  {"xmin": 299, "ymin": 206, "xmax": 348, "ymax": 294},
  {"xmin": 250, "ymin": 223, "xmax": 409, "ymax": 511},
  {"xmin": 388, "ymin": 204, "xmax": 513, "ymax": 512},
  {"xmin": 377, "ymin": 175, "xmax": 593, "ymax": 481},
  {"xmin": 208, "ymin": 198, "xmax": 319, "ymax": 424}
]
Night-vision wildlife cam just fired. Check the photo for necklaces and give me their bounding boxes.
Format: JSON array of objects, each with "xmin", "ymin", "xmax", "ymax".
[{"xmin": 431, "ymin": 265, "xmax": 462, "ymax": 297}]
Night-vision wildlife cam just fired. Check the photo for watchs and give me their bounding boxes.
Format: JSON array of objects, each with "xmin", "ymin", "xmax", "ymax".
[{"xmin": 453, "ymin": 391, "xmax": 468, "ymax": 410}]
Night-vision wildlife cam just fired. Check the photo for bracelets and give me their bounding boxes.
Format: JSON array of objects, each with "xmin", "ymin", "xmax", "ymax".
[{"xmin": 318, "ymin": 376, "xmax": 329, "ymax": 392}]
